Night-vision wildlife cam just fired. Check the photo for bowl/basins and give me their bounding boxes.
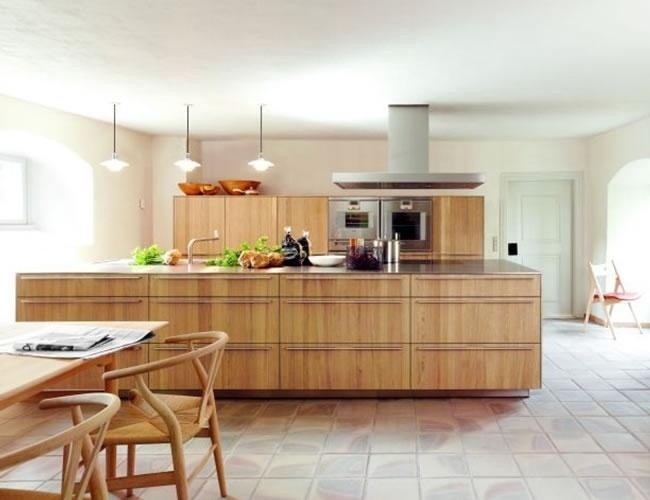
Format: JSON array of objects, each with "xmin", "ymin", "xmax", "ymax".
[
  {"xmin": 178, "ymin": 182, "xmax": 221, "ymax": 196},
  {"xmin": 308, "ymin": 255, "xmax": 346, "ymax": 267},
  {"xmin": 218, "ymin": 179, "xmax": 261, "ymax": 196}
]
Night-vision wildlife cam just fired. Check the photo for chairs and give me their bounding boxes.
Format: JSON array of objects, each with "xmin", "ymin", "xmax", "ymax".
[
  {"xmin": 0, "ymin": 330, "xmax": 230, "ymax": 499},
  {"xmin": 581, "ymin": 259, "xmax": 644, "ymax": 340}
]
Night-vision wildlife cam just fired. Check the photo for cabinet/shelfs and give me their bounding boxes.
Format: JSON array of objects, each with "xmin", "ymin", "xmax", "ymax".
[
  {"xmin": 175, "ymin": 197, "xmax": 328, "ymax": 255},
  {"xmin": 434, "ymin": 197, "xmax": 483, "ymax": 256}
]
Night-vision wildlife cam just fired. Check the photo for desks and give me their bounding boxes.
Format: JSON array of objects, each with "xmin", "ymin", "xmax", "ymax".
[{"xmin": 1, "ymin": 320, "xmax": 171, "ymax": 479}]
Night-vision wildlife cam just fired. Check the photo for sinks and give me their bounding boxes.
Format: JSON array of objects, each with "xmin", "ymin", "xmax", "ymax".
[{"xmin": 177, "ymin": 253, "xmax": 225, "ymax": 265}]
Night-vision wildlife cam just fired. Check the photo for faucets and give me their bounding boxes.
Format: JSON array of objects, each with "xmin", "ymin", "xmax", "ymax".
[{"xmin": 186, "ymin": 228, "xmax": 221, "ymax": 266}]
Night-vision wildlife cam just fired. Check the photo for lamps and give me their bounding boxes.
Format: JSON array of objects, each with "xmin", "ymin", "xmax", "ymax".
[{"xmin": 98, "ymin": 101, "xmax": 276, "ymax": 173}]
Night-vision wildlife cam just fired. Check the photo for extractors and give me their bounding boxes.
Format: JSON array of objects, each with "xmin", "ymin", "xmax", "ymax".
[{"xmin": 331, "ymin": 103, "xmax": 485, "ymax": 191}]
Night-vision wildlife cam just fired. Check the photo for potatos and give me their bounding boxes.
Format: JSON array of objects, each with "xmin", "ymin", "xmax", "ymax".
[
  {"xmin": 164, "ymin": 248, "xmax": 179, "ymax": 265},
  {"xmin": 238, "ymin": 250, "xmax": 283, "ymax": 268}
]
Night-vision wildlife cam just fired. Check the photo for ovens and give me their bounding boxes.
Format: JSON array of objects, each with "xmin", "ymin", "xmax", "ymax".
[{"xmin": 329, "ymin": 199, "xmax": 433, "ymax": 252}]
